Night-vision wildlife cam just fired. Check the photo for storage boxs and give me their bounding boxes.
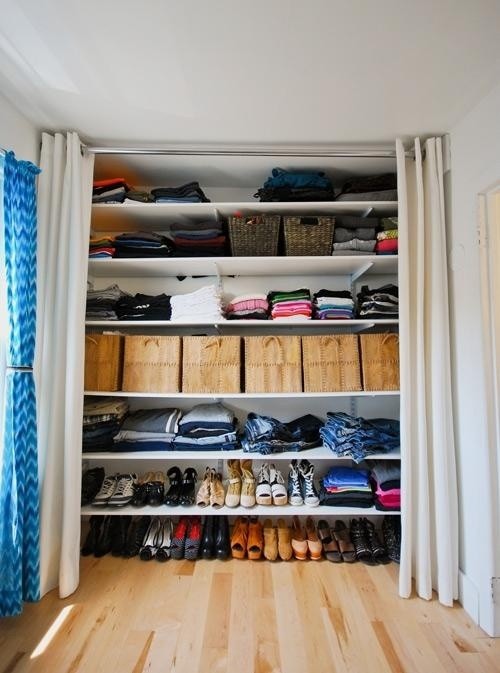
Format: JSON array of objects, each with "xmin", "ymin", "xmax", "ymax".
[
  {"xmin": 121, "ymin": 333, "xmax": 181, "ymax": 394},
  {"xmin": 362, "ymin": 330, "xmax": 400, "ymax": 392},
  {"xmin": 84, "ymin": 334, "xmax": 123, "ymax": 392},
  {"xmin": 181, "ymin": 334, "xmax": 242, "ymax": 395},
  {"xmin": 243, "ymin": 333, "xmax": 302, "ymax": 394},
  {"xmin": 302, "ymin": 332, "xmax": 363, "ymax": 394}
]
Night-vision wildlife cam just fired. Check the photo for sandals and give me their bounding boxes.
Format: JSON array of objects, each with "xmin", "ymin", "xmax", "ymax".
[
  {"xmin": 269, "ymin": 462, "xmax": 288, "ymax": 505},
  {"xmin": 184, "ymin": 515, "xmax": 203, "ymax": 559},
  {"xmin": 230, "ymin": 515, "xmax": 249, "ymax": 558},
  {"xmin": 317, "ymin": 517, "xmax": 389, "ymax": 565},
  {"xmin": 247, "ymin": 514, "xmax": 264, "ymax": 559},
  {"xmin": 164, "ymin": 465, "xmax": 225, "ymax": 509},
  {"xmin": 255, "ymin": 462, "xmax": 272, "ymax": 505},
  {"xmin": 170, "ymin": 515, "xmax": 191, "ymax": 559}
]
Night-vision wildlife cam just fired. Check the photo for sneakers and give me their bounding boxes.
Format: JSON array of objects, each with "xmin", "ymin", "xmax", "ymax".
[
  {"xmin": 225, "ymin": 458, "xmax": 242, "ymax": 508},
  {"xmin": 91, "ymin": 472, "xmax": 120, "ymax": 505},
  {"xmin": 107, "ymin": 472, "xmax": 139, "ymax": 506},
  {"xmin": 240, "ymin": 459, "xmax": 257, "ymax": 506},
  {"xmin": 287, "ymin": 458, "xmax": 304, "ymax": 506},
  {"xmin": 297, "ymin": 458, "xmax": 320, "ymax": 507}
]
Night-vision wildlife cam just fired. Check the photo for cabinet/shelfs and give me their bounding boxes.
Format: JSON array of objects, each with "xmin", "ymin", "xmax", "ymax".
[{"xmin": 79, "ymin": 199, "xmax": 402, "ymax": 519}]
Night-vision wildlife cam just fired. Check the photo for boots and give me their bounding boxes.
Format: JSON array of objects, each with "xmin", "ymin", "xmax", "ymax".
[
  {"xmin": 199, "ymin": 515, "xmax": 216, "ymax": 558},
  {"xmin": 214, "ymin": 515, "xmax": 230, "ymax": 559}
]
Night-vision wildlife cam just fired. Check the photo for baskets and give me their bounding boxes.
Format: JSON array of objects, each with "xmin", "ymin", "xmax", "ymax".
[
  {"xmin": 282, "ymin": 213, "xmax": 336, "ymax": 255},
  {"xmin": 228, "ymin": 214, "xmax": 281, "ymax": 256}
]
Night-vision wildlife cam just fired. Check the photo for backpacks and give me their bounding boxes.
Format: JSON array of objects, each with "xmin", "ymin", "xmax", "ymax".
[{"xmin": 253, "ymin": 167, "xmax": 337, "ymax": 200}]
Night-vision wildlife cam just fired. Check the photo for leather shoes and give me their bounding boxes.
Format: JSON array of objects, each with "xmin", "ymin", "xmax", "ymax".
[
  {"xmin": 131, "ymin": 470, "xmax": 154, "ymax": 506},
  {"xmin": 304, "ymin": 515, "xmax": 323, "ymax": 559},
  {"xmin": 381, "ymin": 519, "xmax": 400, "ymax": 562},
  {"xmin": 291, "ymin": 514, "xmax": 308, "ymax": 560},
  {"xmin": 146, "ymin": 470, "xmax": 164, "ymax": 505},
  {"xmin": 82, "ymin": 514, "xmax": 174, "ymax": 561},
  {"xmin": 263, "ymin": 517, "xmax": 278, "ymax": 560},
  {"xmin": 276, "ymin": 518, "xmax": 293, "ymax": 560}
]
